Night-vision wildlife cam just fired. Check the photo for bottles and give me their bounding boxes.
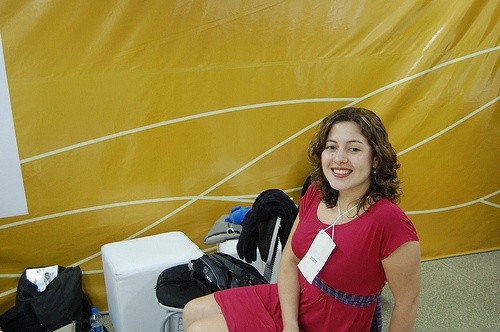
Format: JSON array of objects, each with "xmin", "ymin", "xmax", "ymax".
[{"xmin": 89, "ymin": 308, "xmax": 104, "ymax": 332}]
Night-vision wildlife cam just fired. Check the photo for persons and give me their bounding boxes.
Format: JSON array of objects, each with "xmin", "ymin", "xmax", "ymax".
[{"xmin": 183, "ymin": 107, "xmax": 421, "ymax": 332}]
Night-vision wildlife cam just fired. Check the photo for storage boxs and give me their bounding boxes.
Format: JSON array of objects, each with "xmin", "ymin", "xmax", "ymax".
[{"xmin": 100, "ymin": 231, "xmax": 206, "ymax": 332}]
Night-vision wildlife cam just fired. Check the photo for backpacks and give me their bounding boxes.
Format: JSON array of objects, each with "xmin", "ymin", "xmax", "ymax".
[{"xmin": 188, "ymin": 252, "xmax": 270, "ymax": 295}]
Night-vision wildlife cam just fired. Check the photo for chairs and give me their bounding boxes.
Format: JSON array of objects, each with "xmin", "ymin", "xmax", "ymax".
[{"xmin": 155, "ymin": 188, "xmax": 298, "ymax": 332}]
